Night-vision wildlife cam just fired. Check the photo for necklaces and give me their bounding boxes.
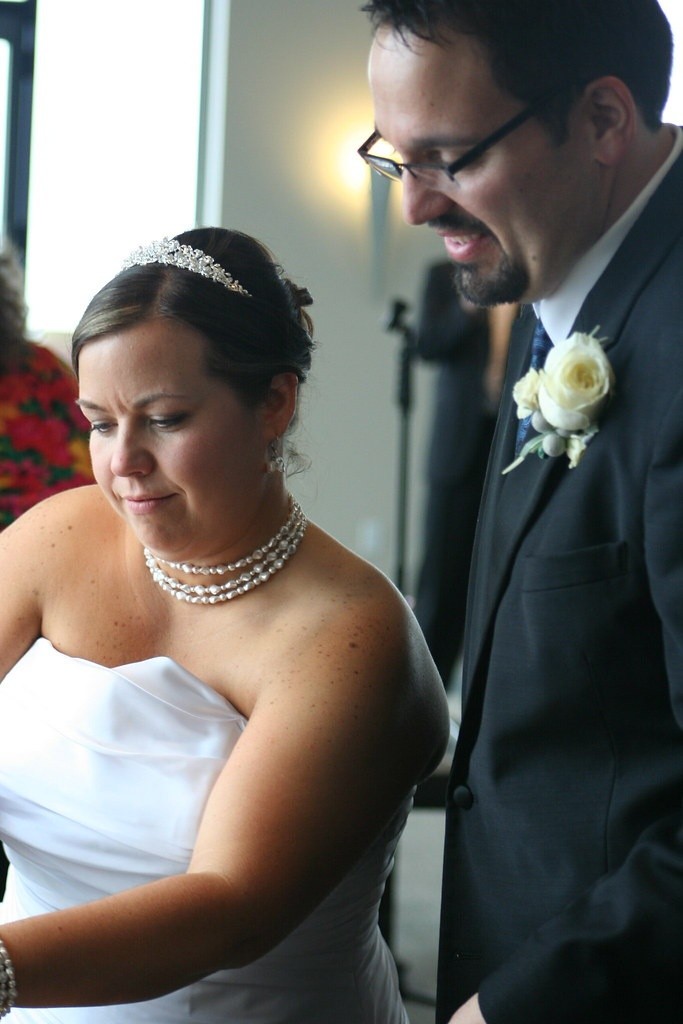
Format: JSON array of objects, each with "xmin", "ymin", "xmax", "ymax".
[{"xmin": 142, "ymin": 499, "xmax": 305, "ymax": 605}]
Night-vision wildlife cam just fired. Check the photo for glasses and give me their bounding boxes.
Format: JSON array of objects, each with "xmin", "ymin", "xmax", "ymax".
[{"xmin": 357, "ymin": 104, "xmax": 540, "ymax": 195}]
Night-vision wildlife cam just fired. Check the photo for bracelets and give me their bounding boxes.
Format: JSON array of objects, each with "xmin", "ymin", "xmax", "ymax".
[{"xmin": 0, "ymin": 937, "xmax": 17, "ymax": 1018}]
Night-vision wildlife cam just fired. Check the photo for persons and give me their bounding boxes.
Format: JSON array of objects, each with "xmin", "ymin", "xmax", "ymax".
[
  {"xmin": 414, "ymin": 264, "xmax": 522, "ymax": 685},
  {"xmin": 350, "ymin": 0, "xmax": 683, "ymax": 1024},
  {"xmin": 0, "ymin": 228, "xmax": 448, "ymax": 1023},
  {"xmin": 0, "ymin": 241, "xmax": 99, "ymax": 533}
]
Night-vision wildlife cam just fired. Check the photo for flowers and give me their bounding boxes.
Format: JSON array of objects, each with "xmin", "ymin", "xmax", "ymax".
[{"xmin": 500, "ymin": 325, "xmax": 617, "ymax": 475}]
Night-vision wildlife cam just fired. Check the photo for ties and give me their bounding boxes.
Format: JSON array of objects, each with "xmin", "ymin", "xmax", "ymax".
[{"xmin": 512, "ymin": 316, "xmax": 555, "ymax": 461}]
